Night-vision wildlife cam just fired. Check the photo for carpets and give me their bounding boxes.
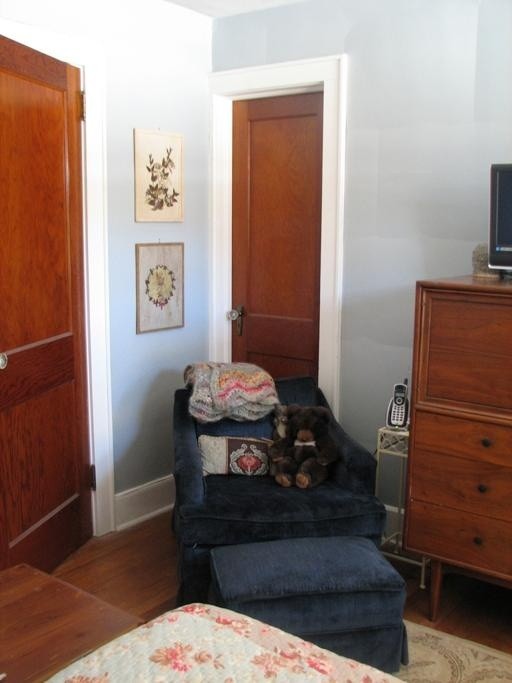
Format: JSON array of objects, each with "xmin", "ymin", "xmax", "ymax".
[{"xmin": 395, "ymin": 613, "xmax": 512, "ymax": 681}]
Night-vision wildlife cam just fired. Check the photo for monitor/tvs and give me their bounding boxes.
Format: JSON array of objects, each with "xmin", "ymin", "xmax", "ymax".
[{"xmin": 488, "ymin": 162, "xmax": 512, "ymax": 272}]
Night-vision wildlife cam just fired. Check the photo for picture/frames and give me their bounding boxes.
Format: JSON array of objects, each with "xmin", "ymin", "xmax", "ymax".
[
  {"xmin": 131, "ymin": 126, "xmax": 188, "ymax": 223},
  {"xmin": 135, "ymin": 242, "xmax": 185, "ymax": 335}
]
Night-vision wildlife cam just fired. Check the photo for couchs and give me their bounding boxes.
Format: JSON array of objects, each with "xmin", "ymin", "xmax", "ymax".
[{"xmin": 164, "ymin": 374, "xmax": 416, "ymax": 673}]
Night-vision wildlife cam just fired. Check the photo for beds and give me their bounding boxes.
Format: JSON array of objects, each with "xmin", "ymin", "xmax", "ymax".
[{"xmin": 35, "ymin": 601, "xmax": 406, "ymax": 683}]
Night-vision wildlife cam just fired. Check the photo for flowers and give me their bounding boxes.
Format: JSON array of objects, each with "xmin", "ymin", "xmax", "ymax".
[{"xmin": 143, "ymin": 145, "xmax": 181, "ymax": 213}]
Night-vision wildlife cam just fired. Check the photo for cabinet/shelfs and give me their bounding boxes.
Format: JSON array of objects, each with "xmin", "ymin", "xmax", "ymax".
[
  {"xmin": 373, "ymin": 424, "xmax": 430, "ymax": 591},
  {"xmin": 402, "ymin": 266, "xmax": 509, "ymax": 621}
]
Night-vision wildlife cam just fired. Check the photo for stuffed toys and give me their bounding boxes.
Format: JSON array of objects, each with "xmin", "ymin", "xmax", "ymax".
[{"xmin": 262, "ymin": 403, "xmax": 340, "ymax": 490}]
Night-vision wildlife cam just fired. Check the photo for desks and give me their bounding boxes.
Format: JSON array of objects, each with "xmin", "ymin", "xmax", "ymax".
[{"xmin": 0, "ymin": 561, "xmax": 145, "ymax": 682}]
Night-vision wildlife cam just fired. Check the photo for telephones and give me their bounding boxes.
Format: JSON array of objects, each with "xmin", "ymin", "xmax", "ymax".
[{"xmin": 386, "ymin": 378, "xmax": 410, "ymax": 432}]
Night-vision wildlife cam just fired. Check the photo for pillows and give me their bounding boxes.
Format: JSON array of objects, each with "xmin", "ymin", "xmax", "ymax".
[{"xmin": 197, "ymin": 433, "xmax": 270, "ymax": 479}]
